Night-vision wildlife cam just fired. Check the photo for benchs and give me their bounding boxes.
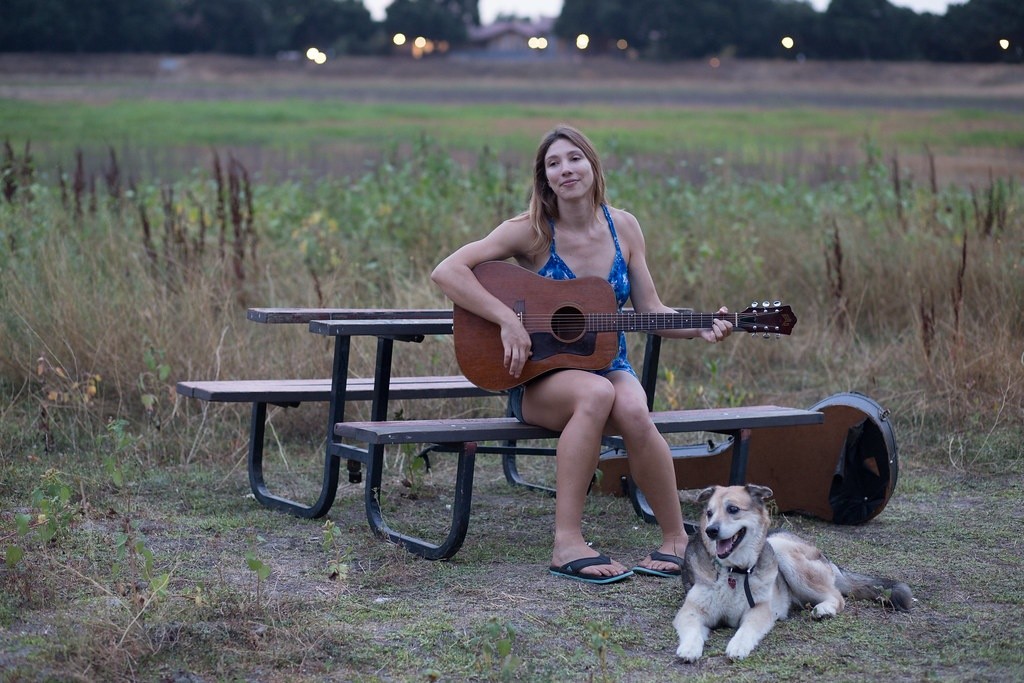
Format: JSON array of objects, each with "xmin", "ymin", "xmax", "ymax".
[
  {"xmin": 176, "ymin": 374, "xmax": 599, "ymax": 517},
  {"xmin": 334, "ymin": 405, "xmax": 826, "ymax": 561}
]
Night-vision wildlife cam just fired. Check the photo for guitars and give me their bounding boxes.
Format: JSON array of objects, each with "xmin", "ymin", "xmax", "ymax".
[{"xmin": 452, "ymin": 261, "xmax": 798, "ymax": 394}]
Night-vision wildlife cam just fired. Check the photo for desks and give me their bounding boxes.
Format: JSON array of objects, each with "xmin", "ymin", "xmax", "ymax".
[{"xmin": 247, "ymin": 308, "xmax": 695, "ymax": 520}]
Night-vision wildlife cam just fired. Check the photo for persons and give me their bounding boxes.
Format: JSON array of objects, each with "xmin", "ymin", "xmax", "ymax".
[{"xmin": 431, "ymin": 123, "xmax": 733, "ymax": 583}]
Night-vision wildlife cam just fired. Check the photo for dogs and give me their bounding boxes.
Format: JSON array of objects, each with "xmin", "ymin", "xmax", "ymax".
[{"xmin": 671, "ymin": 483, "xmax": 913, "ymax": 664}]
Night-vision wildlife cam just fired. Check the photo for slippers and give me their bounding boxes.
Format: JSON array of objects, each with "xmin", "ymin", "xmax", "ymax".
[
  {"xmin": 632, "ymin": 550, "xmax": 683, "ymax": 579},
  {"xmin": 548, "ymin": 553, "xmax": 634, "ymax": 584}
]
